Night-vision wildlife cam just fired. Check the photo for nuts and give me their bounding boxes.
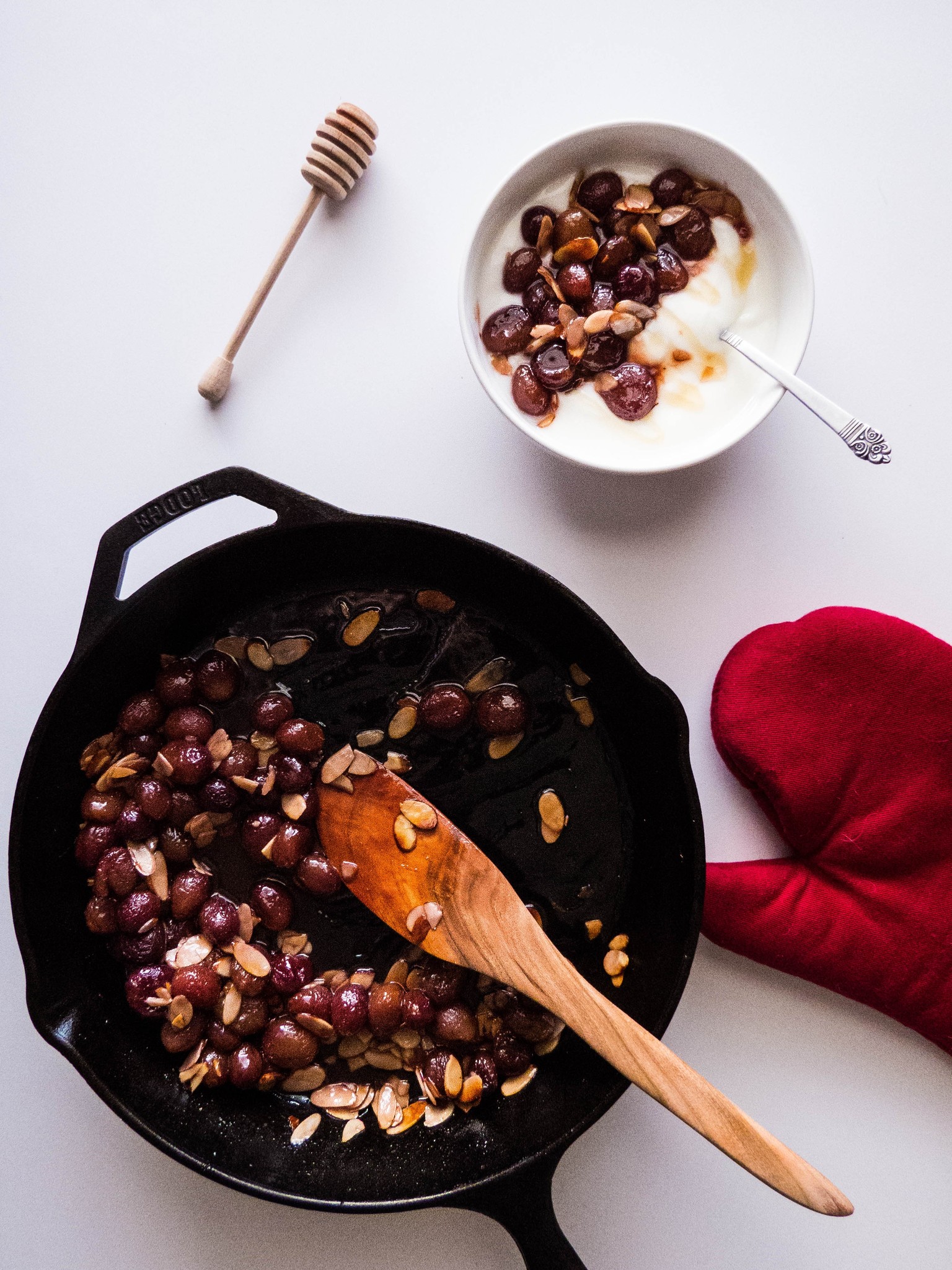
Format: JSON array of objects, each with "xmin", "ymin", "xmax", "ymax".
[
  {"xmin": 70, "ymin": 588, "xmax": 629, "ymax": 1150},
  {"xmin": 482, "ymin": 169, "xmax": 731, "ymax": 419}
]
